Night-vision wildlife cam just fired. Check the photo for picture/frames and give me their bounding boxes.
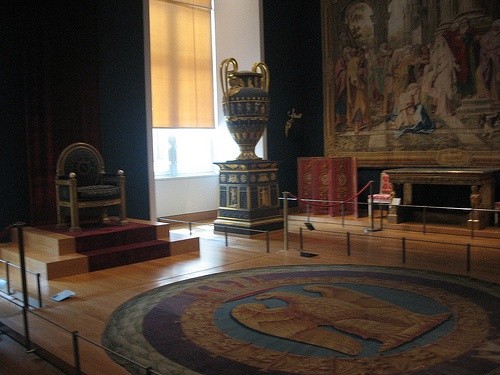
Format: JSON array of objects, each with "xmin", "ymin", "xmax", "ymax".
[{"xmin": 320, "ymin": 0, "xmax": 500, "ymax": 168}]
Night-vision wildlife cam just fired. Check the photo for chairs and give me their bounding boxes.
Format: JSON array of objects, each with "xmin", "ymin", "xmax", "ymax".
[
  {"xmin": 367, "ymin": 170, "xmax": 394, "ymax": 229},
  {"xmin": 495, "ymin": 202, "xmax": 500, "ymax": 224},
  {"xmin": 55, "ymin": 143, "xmax": 128, "ymax": 232}
]
